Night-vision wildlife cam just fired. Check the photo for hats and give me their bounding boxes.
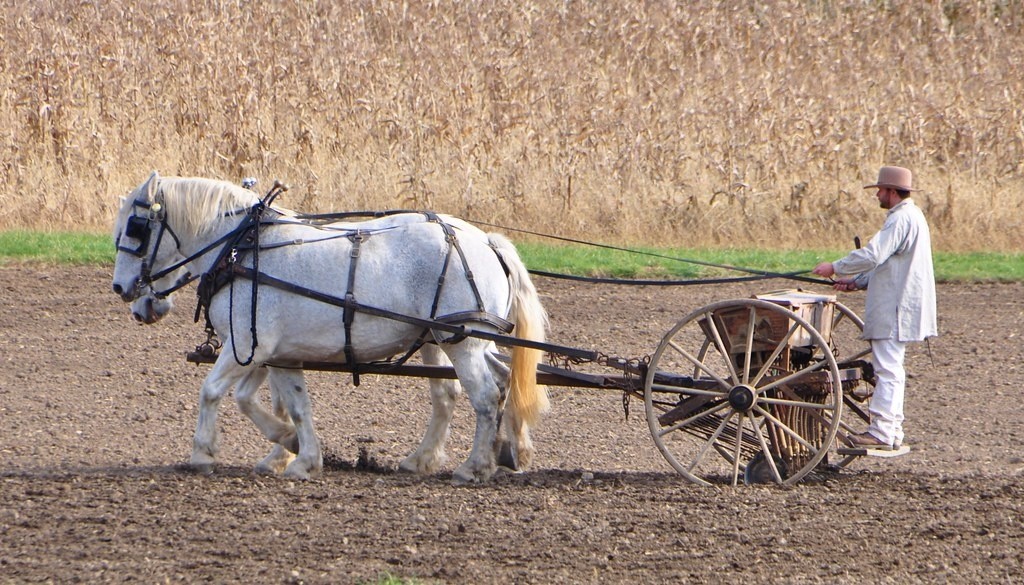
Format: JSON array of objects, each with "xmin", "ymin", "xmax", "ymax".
[{"xmin": 863, "ymin": 166, "xmax": 924, "ymax": 192}]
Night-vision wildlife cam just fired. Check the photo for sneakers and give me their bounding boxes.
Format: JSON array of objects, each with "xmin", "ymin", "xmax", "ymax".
[{"xmin": 848, "ymin": 432, "xmax": 900, "ymax": 451}]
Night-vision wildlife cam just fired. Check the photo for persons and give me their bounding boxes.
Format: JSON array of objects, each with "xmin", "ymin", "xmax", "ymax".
[{"xmin": 812, "ymin": 166, "xmax": 937, "ymax": 451}]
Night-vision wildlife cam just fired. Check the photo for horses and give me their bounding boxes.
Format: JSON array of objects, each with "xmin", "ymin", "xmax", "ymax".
[{"xmin": 112, "ymin": 170, "xmax": 552, "ymax": 485}]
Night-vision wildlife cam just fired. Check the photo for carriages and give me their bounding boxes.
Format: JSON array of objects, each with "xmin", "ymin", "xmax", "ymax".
[{"xmin": 112, "ymin": 169, "xmax": 875, "ymax": 490}]
